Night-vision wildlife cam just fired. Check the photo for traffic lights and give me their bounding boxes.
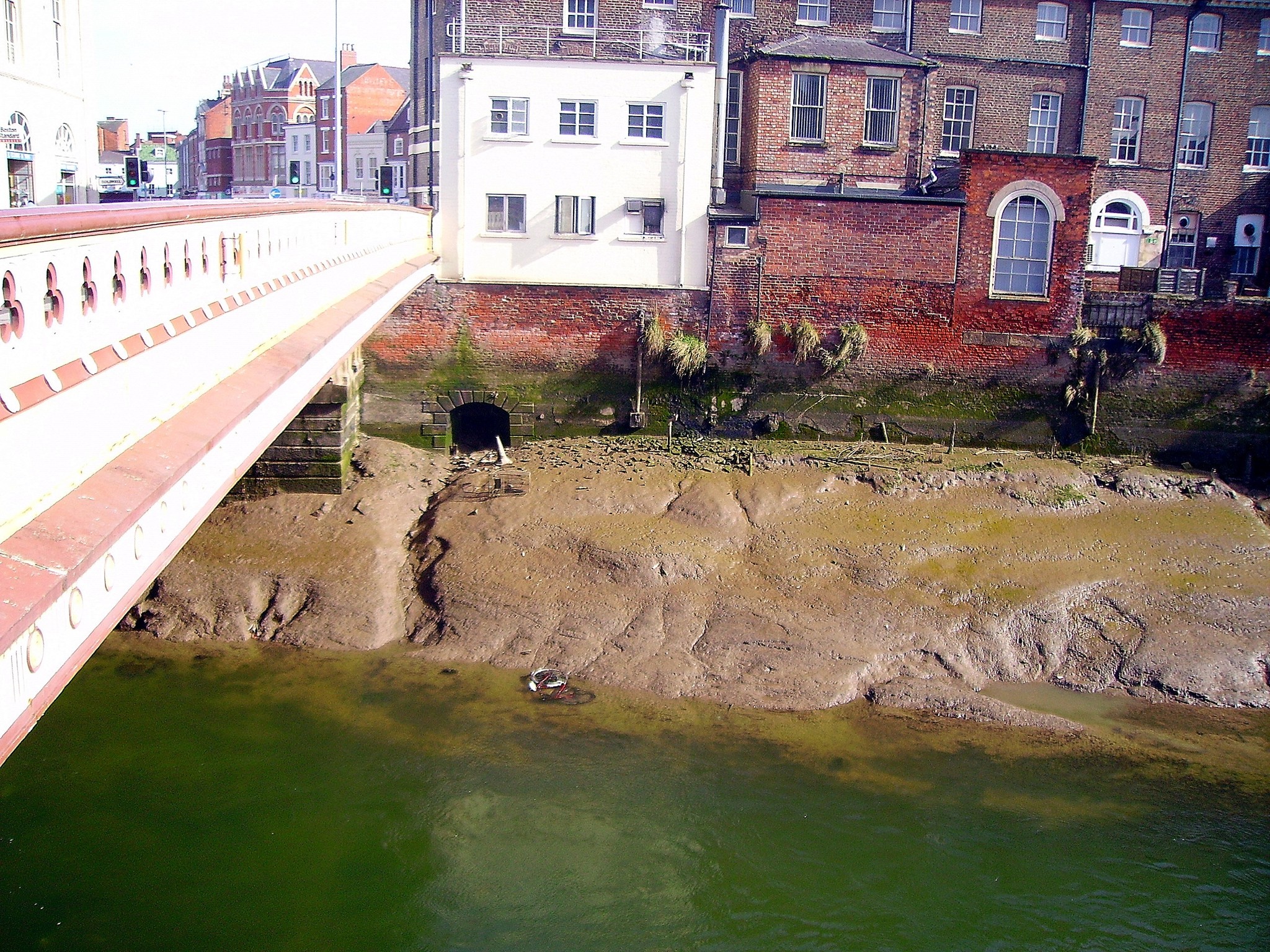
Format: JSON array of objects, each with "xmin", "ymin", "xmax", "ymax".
[
  {"xmin": 289, "ymin": 161, "xmax": 300, "ymax": 185},
  {"xmin": 375, "ymin": 165, "xmax": 394, "ymax": 197},
  {"xmin": 125, "ymin": 157, "xmax": 139, "ymax": 189}
]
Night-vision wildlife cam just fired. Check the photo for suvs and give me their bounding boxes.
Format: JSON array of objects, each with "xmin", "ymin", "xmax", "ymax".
[{"xmin": 179, "ymin": 188, "xmax": 191, "ymax": 199}]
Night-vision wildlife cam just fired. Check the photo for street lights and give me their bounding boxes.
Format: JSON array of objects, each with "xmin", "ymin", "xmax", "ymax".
[{"xmin": 157, "ymin": 109, "xmax": 168, "ymax": 187}]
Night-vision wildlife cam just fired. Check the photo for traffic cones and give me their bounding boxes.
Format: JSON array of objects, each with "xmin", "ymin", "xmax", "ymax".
[{"xmin": 494, "ymin": 434, "xmax": 514, "ymax": 466}]
